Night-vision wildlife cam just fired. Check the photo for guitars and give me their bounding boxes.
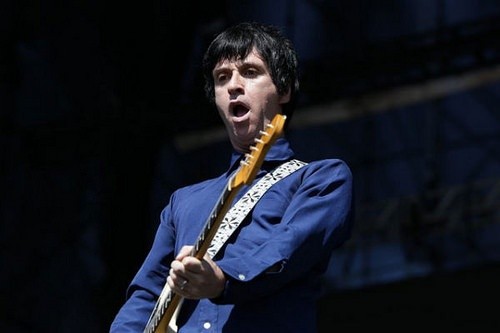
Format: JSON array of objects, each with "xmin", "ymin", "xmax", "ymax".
[{"xmin": 142, "ymin": 114, "xmax": 287, "ymax": 333}]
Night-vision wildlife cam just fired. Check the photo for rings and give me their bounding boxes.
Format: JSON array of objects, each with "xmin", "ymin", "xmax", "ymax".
[{"xmin": 180, "ymin": 280, "xmax": 190, "ymax": 292}]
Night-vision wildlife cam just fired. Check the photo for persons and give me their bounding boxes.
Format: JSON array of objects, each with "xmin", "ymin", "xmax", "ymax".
[{"xmin": 108, "ymin": 22, "xmax": 353, "ymax": 333}]
